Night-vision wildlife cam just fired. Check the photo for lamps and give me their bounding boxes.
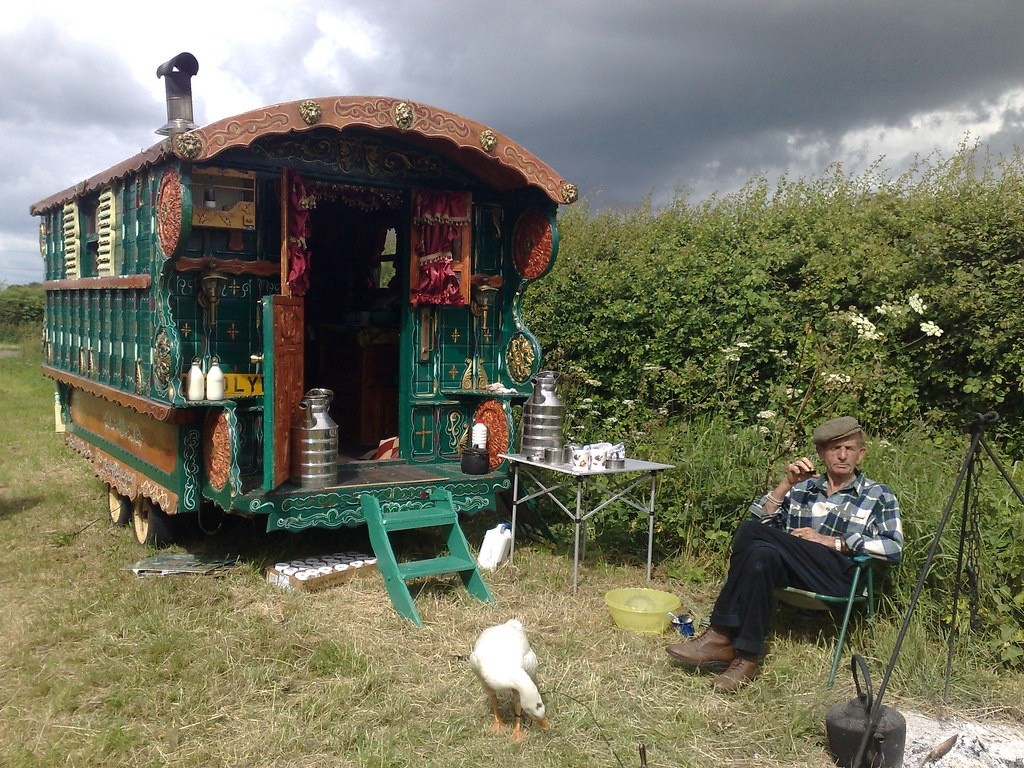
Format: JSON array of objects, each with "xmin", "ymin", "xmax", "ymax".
[
  {"xmin": 199, "ymin": 261, "xmax": 227, "ymax": 326},
  {"xmin": 473, "ymin": 276, "xmax": 499, "ymax": 330}
]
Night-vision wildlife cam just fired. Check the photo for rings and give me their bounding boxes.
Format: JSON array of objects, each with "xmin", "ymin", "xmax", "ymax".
[{"xmin": 799, "ymin": 533, "xmax": 802, "ymax": 538}]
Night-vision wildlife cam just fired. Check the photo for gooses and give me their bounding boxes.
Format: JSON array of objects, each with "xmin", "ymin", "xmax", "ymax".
[{"xmin": 470, "ymin": 619, "xmax": 550, "ymax": 742}]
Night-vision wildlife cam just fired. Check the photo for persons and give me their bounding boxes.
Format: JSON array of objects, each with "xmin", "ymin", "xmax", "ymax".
[{"xmin": 665, "ymin": 416, "xmax": 904, "ymax": 694}]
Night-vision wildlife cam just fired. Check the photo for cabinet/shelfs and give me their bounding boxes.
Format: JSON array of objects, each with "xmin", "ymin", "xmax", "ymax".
[{"xmin": 303, "ymin": 317, "xmax": 400, "ymax": 446}]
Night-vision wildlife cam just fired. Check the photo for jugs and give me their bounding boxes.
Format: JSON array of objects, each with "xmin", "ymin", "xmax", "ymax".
[
  {"xmin": 521, "ymin": 371, "xmax": 565, "ymax": 459},
  {"xmin": 290, "ymin": 387, "xmax": 339, "ymax": 488}
]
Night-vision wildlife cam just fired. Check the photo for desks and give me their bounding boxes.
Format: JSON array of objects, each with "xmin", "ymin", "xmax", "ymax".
[{"xmin": 496, "ymin": 452, "xmax": 675, "ymax": 595}]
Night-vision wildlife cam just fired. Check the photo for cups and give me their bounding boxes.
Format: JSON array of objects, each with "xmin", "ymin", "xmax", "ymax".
[
  {"xmin": 545, "ymin": 442, "xmax": 626, "ymax": 471},
  {"xmin": 671, "ymin": 613, "xmax": 696, "ymax": 637}
]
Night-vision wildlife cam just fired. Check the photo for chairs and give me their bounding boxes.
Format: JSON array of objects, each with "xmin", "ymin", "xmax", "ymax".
[{"xmin": 772, "ymin": 556, "xmax": 895, "ymax": 692}]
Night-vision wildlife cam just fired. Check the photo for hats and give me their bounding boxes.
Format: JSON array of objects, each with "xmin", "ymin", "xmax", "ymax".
[{"xmin": 812, "ymin": 416, "xmax": 862, "ymax": 445}]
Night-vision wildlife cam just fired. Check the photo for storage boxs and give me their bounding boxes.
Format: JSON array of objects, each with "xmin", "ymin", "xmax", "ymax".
[{"xmin": 266, "ymin": 550, "xmax": 377, "ymax": 593}]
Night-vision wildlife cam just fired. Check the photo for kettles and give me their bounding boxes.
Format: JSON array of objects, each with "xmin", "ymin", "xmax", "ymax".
[
  {"xmin": 826, "ymin": 653, "xmax": 906, "ymax": 768},
  {"xmin": 459, "ymin": 443, "xmax": 489, "ymax": 474}
]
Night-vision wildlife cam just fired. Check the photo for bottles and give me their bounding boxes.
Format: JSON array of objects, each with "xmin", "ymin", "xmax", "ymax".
[
  {"xmin": 472, "ymin": 420, "xmax": 487, "ymax": 449},
  {"xmin": 207, "ymin": 363, "xmax": 224, "ymax": 401},
  {"xmin": 186, "ymin": 362, "xmax": 205, "ymax": 401}
]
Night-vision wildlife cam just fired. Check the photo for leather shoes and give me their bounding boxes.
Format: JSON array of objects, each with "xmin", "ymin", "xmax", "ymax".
[
  {"xmin": 712, "ymin": 656, "xmax": 761, "ymax": 695},
  {"xmin": 666, "ymin": 625, "xmax": 734, "ymax": 666}
]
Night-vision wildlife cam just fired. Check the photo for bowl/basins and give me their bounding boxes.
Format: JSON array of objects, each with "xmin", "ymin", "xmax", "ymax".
[{"xmin": 604, "ymin": 588, "xmax": 681, "ymax": 634}]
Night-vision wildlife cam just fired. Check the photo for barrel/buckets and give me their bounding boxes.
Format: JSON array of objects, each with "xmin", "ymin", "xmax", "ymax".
[{"xmin": 478, "ymin": 523, "xmax": 511, "ymax": 570}]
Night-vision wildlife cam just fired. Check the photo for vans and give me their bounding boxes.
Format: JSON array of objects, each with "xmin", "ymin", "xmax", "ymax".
[{"xmin": 28, "ymin": 51, "xmax": 578, "ymax": 627}]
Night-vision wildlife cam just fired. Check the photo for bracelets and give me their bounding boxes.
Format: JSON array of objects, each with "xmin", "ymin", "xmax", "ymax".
[
  {"xmin": 766, "ymin": 491, "xmax": 784, "ymax": 506},
  {"xmin": 834, "ymin": 535, "xmax": 848, "ymax": 556}
]
